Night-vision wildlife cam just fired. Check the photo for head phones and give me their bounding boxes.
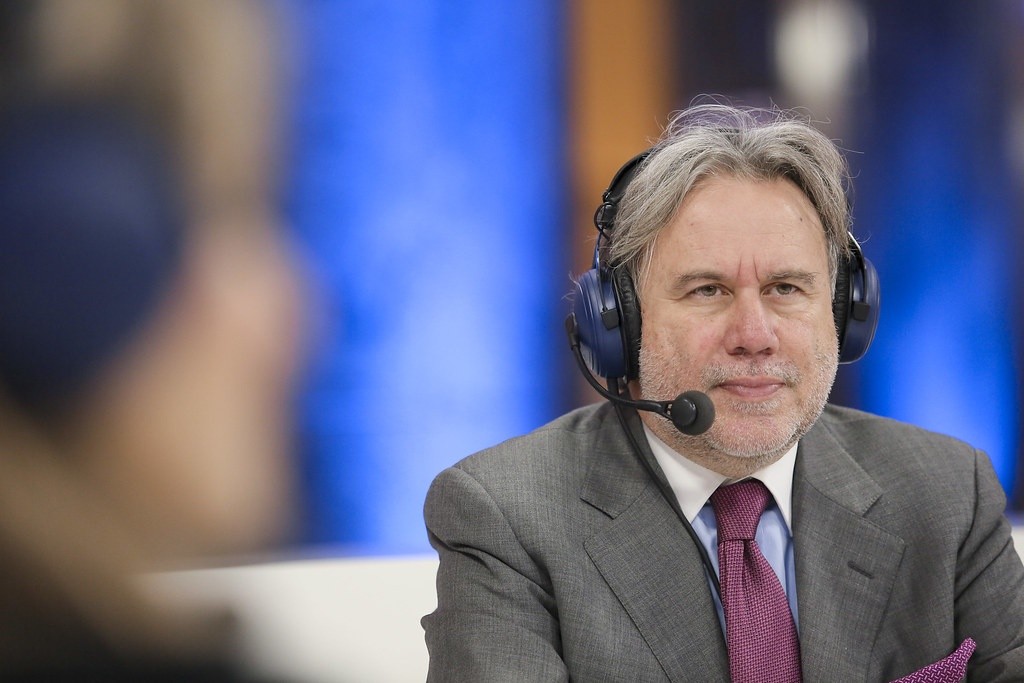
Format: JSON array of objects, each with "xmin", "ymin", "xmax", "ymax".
[
  {"xmin": 0, "ymin": 0, "xmax": 183, "ymax": 415},
  {"xmin": 571, "ymin": 147, "xmax": 879, "ymax": 390}
]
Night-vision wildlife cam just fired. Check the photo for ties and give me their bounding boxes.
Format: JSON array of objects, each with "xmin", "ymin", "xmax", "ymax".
[{"xmin": 710, "ymin": 480, "xmax": 803, "ymax": 683}]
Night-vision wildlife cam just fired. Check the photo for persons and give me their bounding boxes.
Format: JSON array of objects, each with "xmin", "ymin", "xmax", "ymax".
[
  {"xmin": 420, "ymin": 101, "xmax": 1024, "ymax": 683},
  {"xmin": 0, "ymin": 62, "xmax": 322, "ymax": 683}
]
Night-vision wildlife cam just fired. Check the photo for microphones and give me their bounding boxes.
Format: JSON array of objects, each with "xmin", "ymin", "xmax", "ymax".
[{"xmin": 565, "ymin": 313, "xmax": 716, "ymax": 435}]
